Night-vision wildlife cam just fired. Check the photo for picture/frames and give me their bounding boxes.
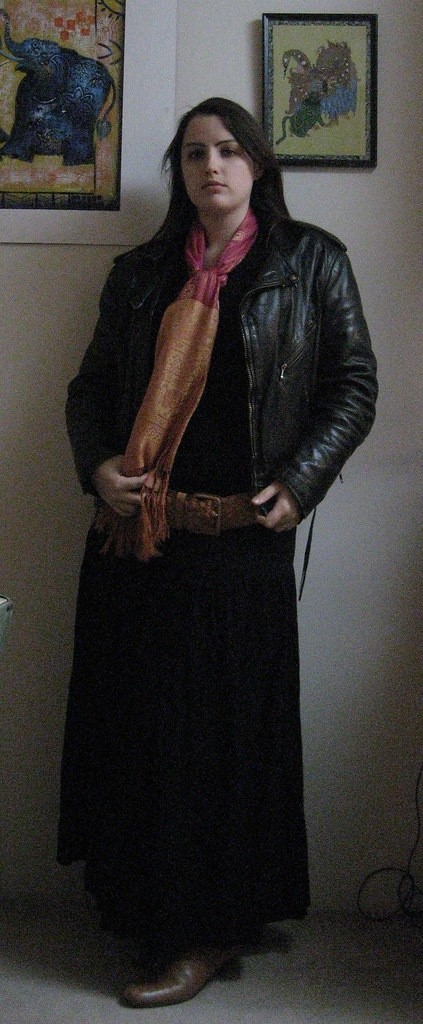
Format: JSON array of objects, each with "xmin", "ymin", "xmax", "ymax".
[{"xmin": 263, "ymin": 12, "xmax": 378, "ymax": 168}]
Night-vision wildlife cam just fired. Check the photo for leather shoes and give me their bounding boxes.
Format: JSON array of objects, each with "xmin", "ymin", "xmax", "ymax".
[{"xmin": 122, "ymin": 937, "xmax": 229, "ymax": 1008}]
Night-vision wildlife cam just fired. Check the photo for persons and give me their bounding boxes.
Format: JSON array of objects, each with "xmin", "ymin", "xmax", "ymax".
[{"xmin": 55, "ymin": 98, "xmax": 379, "ymax": 1008}]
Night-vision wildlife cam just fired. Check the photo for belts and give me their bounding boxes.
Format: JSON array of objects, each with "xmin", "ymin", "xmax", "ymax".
[{"xmin": 164, "ymin": 489, "xmax": 260, "ymax": 536}]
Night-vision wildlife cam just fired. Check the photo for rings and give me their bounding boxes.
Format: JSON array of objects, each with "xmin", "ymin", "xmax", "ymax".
[{"xmin": 283, "ymin": 524, "xmax": 287, "ymax": 528}]
